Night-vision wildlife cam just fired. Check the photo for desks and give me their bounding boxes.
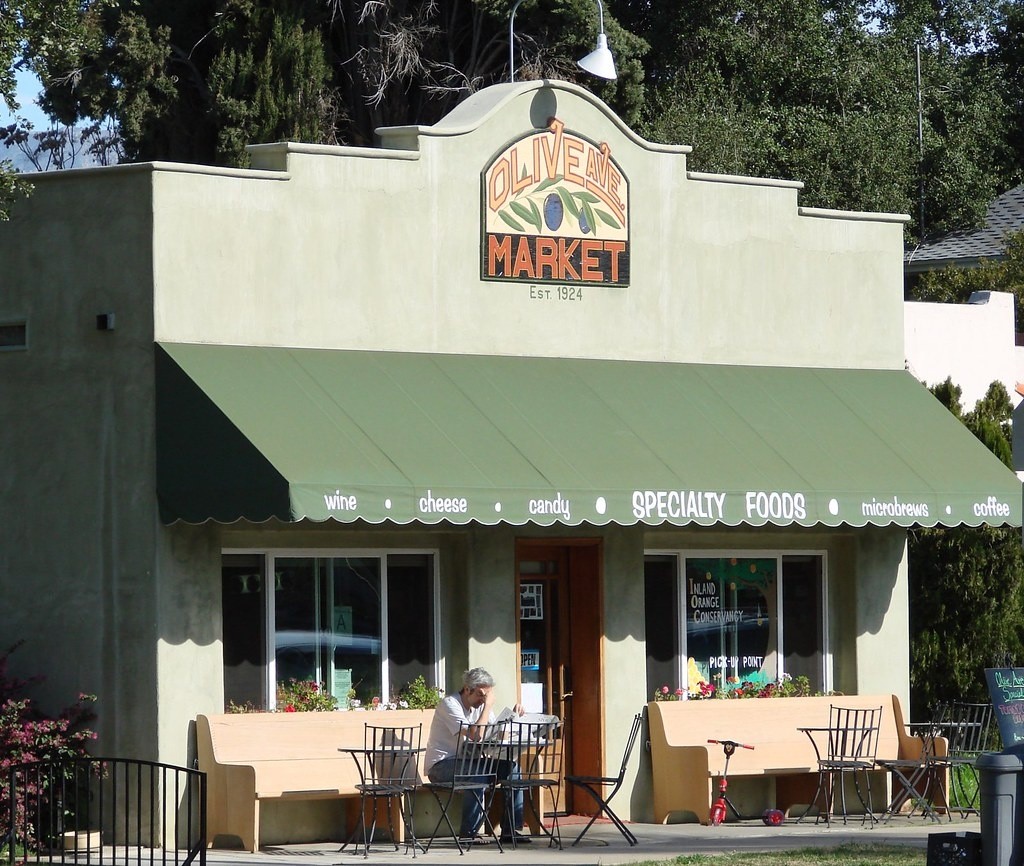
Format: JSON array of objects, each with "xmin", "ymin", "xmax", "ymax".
[
  {"xmin": 336, "ymin": 746, "xmax": 427, "ymax": 854},
  {"xmin": 470, "ymin": 740, "xmax": 560, "ymax": 849},
  {"xmin": 903, "ymin": 720, "xmax": 984, "ymax": 820},
  {"xmin": 795, "ymin": 727, "xmax": 880, "ymax": 825}
]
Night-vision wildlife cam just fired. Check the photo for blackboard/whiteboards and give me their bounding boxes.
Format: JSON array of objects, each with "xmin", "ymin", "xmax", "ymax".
[{"xmin": 983, "ymin": 662, "xmax": 1024, "ymax": 760}]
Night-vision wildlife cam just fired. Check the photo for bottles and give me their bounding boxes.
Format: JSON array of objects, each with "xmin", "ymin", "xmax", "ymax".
[{"xmin": 276, "ymin": 680, "xmax": 286, "ymax": 712}]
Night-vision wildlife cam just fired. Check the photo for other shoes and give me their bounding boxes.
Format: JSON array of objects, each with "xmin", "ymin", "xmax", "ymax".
[
  {"xmin": 499, "ymin": 831, "xmax": 532, "ymax": 843},
  {"xmin": 458, "ymin": 834, "xmax": 490, "ymax": 845}
]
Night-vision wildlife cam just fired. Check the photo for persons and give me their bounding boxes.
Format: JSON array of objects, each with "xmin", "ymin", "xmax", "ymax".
[{"xmin": 424, "ymin": 667, "xmax": 532, "ymax": 845}]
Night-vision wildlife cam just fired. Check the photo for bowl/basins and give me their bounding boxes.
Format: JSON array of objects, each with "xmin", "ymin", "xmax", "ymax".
[{"xmin": 60, "ymin": 828, "xmax": 102, "ymax": 848}]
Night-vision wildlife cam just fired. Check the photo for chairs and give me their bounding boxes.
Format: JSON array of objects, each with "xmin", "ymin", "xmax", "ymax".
[
  {"xmin": 871, "ymin": 699, "xmax": 996, "ymax": 828},
  {"xmin": 818, "ymin": 704, "xmax": 882, "ymax": 829},
  {"xmin": 421, "ymin": 721, "xmax": 510, "ymax": 855},
  {"xmin": 354, "ymin": 721, "xmax": 428, "ymax": 860},
  {"xmin": 565, "ymin": 713, "xmax": 646, "ymax": 847},
  {"xmin": 496, "ymin": 719, "xmax": 568, "ymax": 849}
]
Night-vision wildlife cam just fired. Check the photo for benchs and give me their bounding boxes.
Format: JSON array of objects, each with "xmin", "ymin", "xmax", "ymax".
[
  {"xmin": 648, "ymin": 694, "xmax": 951, "ymax": 825},
  {"xmin": 197, "ymin": 708, "xmax": 546, "ymax": 856}
]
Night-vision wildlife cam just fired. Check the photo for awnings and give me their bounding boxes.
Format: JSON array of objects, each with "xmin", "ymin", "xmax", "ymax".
[{"xmin": 151, "ymin": 342, "xmax": 1024, "ymax": 527}]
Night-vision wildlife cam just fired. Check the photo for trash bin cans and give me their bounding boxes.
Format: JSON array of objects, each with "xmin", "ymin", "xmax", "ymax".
[{"xmin": 972, "ymin": 750, "xmax": 1024, "ymax": 866}]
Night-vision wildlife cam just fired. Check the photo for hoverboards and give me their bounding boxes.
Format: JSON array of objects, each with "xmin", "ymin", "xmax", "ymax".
[{"xmin": 706, "ymin": 740, "xmax": 784, "ymax": 827}]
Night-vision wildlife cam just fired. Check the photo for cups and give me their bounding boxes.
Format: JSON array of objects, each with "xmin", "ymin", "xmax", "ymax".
[{"xmin": 498, "ymin": 723, "xmax": 508, "ymax": 744}]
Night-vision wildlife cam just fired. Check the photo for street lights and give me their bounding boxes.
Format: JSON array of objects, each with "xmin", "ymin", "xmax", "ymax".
[{"xmin": 918, "ymin": 85, "xmax": 953, "ymax": 238}]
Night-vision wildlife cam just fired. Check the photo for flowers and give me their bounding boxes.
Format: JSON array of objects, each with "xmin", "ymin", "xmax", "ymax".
[
  {"xmin": 653, "ymin": 672, "xmax": 811, "ymax": 701},
  {"xmin": 275, "ymin": 675, "xmax": 380, "ymax": 712}
]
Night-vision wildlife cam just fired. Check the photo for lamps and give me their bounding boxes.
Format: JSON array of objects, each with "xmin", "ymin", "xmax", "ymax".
[{"xmin": 511, "ymin": 0, "xmax": 618, "ymax": 83}]
